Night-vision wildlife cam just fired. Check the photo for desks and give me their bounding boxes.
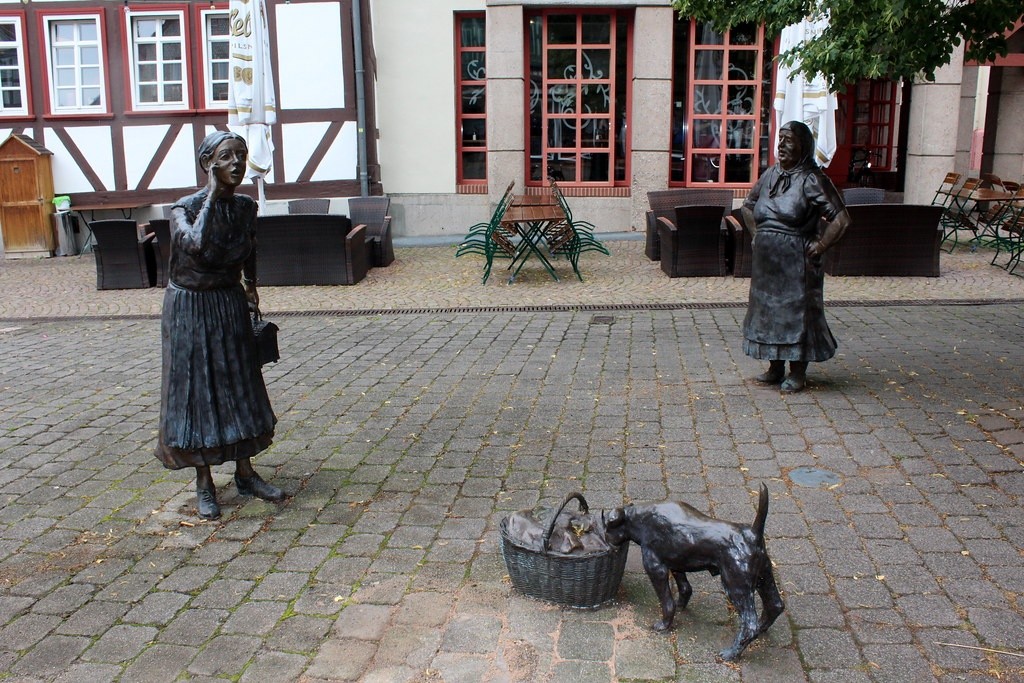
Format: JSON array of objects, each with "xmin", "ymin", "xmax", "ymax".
[
  {"xmin": 66, "ymin": 200, "xmax": 150, "ymax": 260},
  {"xmin": 941, "ymin": 187, "xmax": 1024, "ymax": 244},
  {"xmin": 511, "ymin": 193, "xmax": 559, "ymax": 260},
  {"xmin": 502, "ymin": 206, "xmax": 562, "ymax": 284}
]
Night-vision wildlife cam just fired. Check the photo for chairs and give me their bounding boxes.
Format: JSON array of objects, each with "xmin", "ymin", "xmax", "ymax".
[
  {"xmin": 456, "ymin": 175, "xmax": 613, "ymax": 287},
  {"xmin": 926, "ymin": 171, "xmax": 1024, "ymax": 280}
]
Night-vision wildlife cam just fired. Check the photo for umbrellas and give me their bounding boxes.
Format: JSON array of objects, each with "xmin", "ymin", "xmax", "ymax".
[
  {"xmin": 773, "ymin": 0, "xmax": 840, "ymax": 169},
  {"xmin": 695, "ymin": 18, "xmax": 729, "ymax": 160},
  {"xmin": 226, "ymin": 0, "xmax": 279, "ymax": 215}
]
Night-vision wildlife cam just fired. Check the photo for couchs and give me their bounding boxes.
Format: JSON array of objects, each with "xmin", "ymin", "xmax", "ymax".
[
  {"xmin": 842, "ymin": 185, "xmax": 888, "ymax": 204},
  {"xmin": 346, "ymin": 196, "xmax": 398, "ymax": 265},
  {"xmin": 87, "ymin": 218, "xmax": 155, "ymax": 292},
  {"xmin": 148, "ymin": 218, "xmax": 170, "ymax": 287},
  {"xmin": 656, "ymin": 203, "xmax": 745, "ymax": 278},
  {"xmin": 643, "ymin": 188, "xmax": 735, "ymax": 260},
  {"xmin": 250, "ymin": 214, "xmax": 371, "ymax": 287},
  {"xmin": 824, "ymin": 203, "xmax": 946, "ymax": 279},
  {"xmin": 286, "ymin": 198, "xmax": 330, "ymax": 213}
]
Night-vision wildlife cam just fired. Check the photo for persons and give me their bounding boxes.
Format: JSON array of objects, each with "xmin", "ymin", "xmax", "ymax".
[
  {"xmin": 153, "ymin": 131, "xmax": 286, "ymax": 517},
  {"xmin": 740, "ymin": 121, "xmax": 850, "ymax": 393}
]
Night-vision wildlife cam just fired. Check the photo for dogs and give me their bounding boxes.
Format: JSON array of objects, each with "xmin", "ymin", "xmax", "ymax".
[{"xmin": 605, "ymin": 484, "xmax": 785, "ymax": 663}]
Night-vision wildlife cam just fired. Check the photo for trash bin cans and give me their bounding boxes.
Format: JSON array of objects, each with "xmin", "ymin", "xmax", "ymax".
[{"xmin": 50, "ymin": 211, "xmax": 79, "ymax": 256}]
